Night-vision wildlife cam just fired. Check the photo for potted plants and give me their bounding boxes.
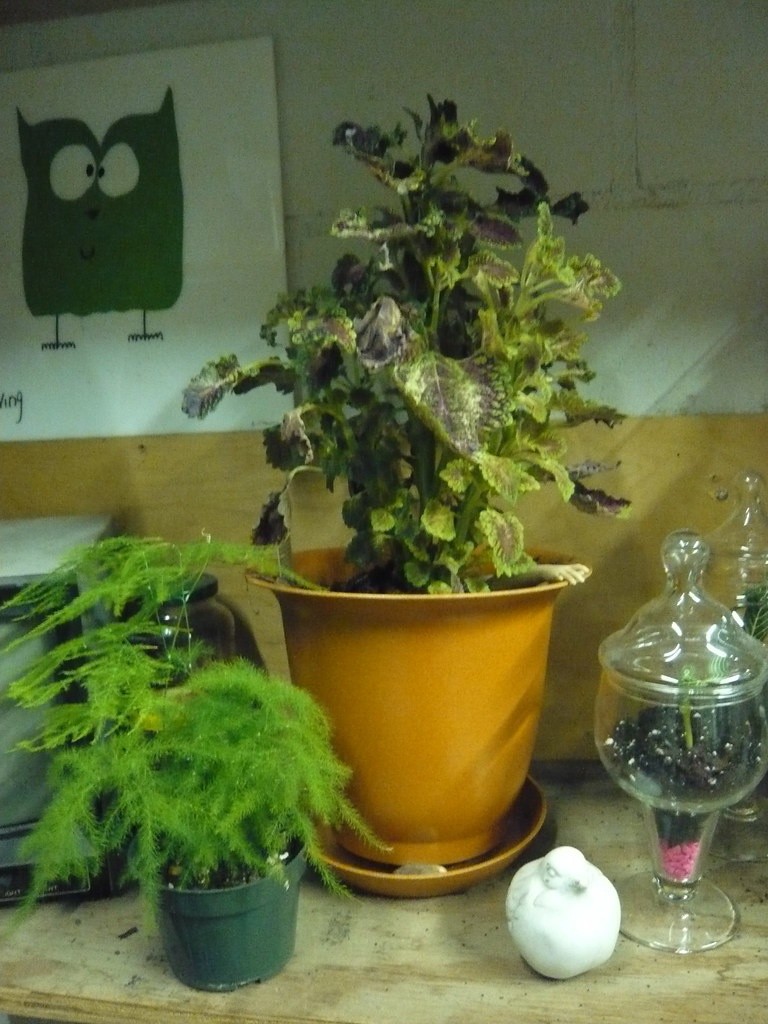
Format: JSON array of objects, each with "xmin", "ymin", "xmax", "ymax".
[
  {"xmin": 180, "ymin": 94, "xmax": 635, "ymax": 866},
  {"xmin": 0, "ymin": 535, "xmax": 397, "ymax": 994}
]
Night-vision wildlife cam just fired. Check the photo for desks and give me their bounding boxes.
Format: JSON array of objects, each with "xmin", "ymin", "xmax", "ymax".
[{"xmin": 0, "ymin": 782, "xmax": 768, "ymax": 1024}]
